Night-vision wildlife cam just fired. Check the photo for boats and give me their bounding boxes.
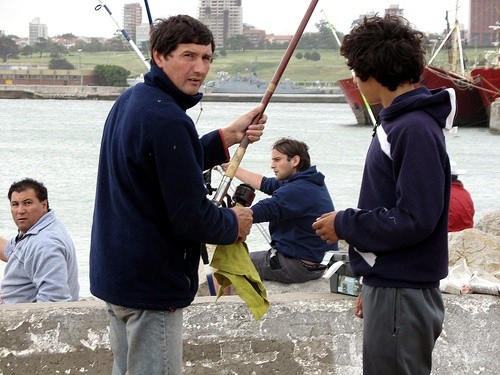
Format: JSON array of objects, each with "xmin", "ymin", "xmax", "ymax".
[
  {"xmin": 471, "ymin": 21, "xmax": 500, "ymax": 126},
  {"xmin": 336, "ymin": 19, "xmax": 489, "ymax": 126}
]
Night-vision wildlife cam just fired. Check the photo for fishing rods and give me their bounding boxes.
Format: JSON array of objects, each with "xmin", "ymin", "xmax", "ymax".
[
  {"xmin": 314, "ymin": 0, "xmax": 377, "ymax": 126},
  {"xmin": 96, "ymin": 0, "xmax": 274, "ymax": 246},
  {"xmin": 212, "ymin": 0, "xmax": 317, "ymax": 210}
]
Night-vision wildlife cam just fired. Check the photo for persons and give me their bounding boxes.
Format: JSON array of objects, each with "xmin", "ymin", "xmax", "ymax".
[
  {"xmin": 311, "ymin": 13, "xmax": 457, "ymax": 374},
  {"xmin": 0, "ymin": 177, "xmax": 82, "ymax": 303},
  {"xmin": 447, "ymin": 170, "xmax": 475, "ymax": 233},
  {"xmin": 88, "ymin": 15, "xmax": 268, "ymax": 375},
  {"xmin": 211, "ymin": 137, "xmax": 339, "ymax": 297}
]
